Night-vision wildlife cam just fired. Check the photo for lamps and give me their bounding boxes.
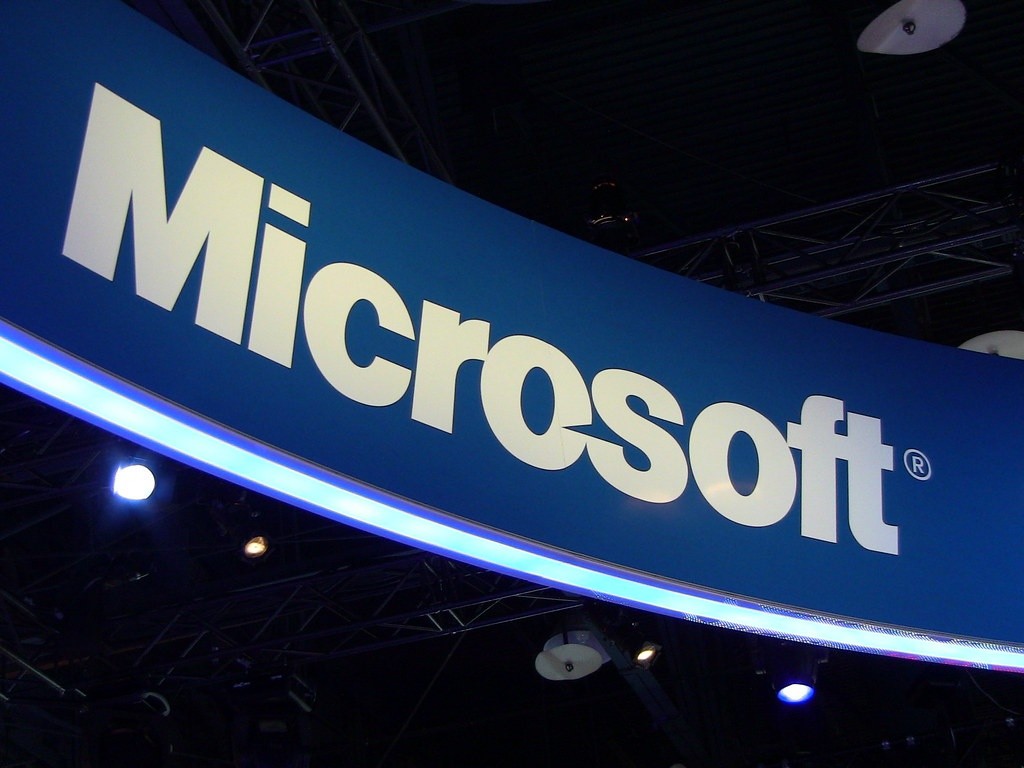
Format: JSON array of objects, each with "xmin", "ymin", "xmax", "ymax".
[
  {"xmin": 212, "ymin": 501, "xmax": 276, "ymax": 562},
  {"xmin": 602, "ymin": 614, "xmax": 665, "ymax": 667},
  {"xmin": 767, "ymin": 644, "xmax": 815, "ymax": 703},
  {"xmin": 577, "ymin": 176, "xmax": 648, "ymax": 249},
  {"xmin": 714, "ymin": 235, "xmax": 767, "ymax": 290}
]
